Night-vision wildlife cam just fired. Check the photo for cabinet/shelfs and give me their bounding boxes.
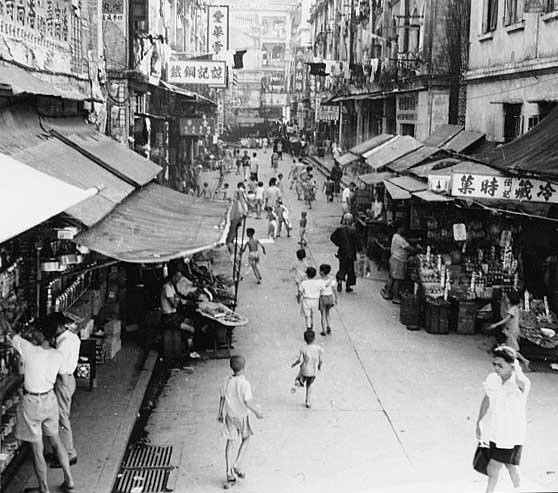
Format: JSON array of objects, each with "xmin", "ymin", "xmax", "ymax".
[
  {"xmin": 408, "ymin": 254, "xmax": 520, "ymax": 335},
  {"xmin": 0, "ymin": 375, "xmax": 32, "ymax": 492}
]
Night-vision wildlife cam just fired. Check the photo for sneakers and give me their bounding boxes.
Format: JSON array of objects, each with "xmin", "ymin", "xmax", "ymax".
[
  {"xmin": 336, "ymin": 280, "xmax": 354, "ymax": 293},
  {"xmin": 44, "ymin": 452, "xmax": 77, "ymax": 468},
  {"xmin": 320, "ymin": 326, "xmax": 331, "ymax": 336},
  {"xmin": 24, "ymin": 487, "xmax": 51, "ymax": 493},
  {"xmin": 57, "ymin": 481, "xmax": 75, "ymax": 493}
]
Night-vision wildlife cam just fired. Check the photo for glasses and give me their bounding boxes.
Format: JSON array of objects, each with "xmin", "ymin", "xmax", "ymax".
[
  {"xmin": 25, "ymin": 388, "xmax": 54, "ymax": 396},
  {"xmin": 492, "ymin": 362, "xmax": 503, "ymax": 370}
]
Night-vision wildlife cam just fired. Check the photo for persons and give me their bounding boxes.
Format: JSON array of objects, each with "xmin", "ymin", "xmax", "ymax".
[
  {"xmin": 487, "ymin": 291, "xmax": 530, "ymax": 371},
  {"xmin": 475, "ymin": 346, "xmax": 531, "ymax": 493},
  {"xmin": 45, "ymin": 312, "xmax": 81, "ymax": 468},
  {"xmin": 157, "ymin": 119, "xmax": 422, "ymax": 489},
  {"xmin": 0, "ymin": 306, "xmax": 76, "ymax": 493}
]
